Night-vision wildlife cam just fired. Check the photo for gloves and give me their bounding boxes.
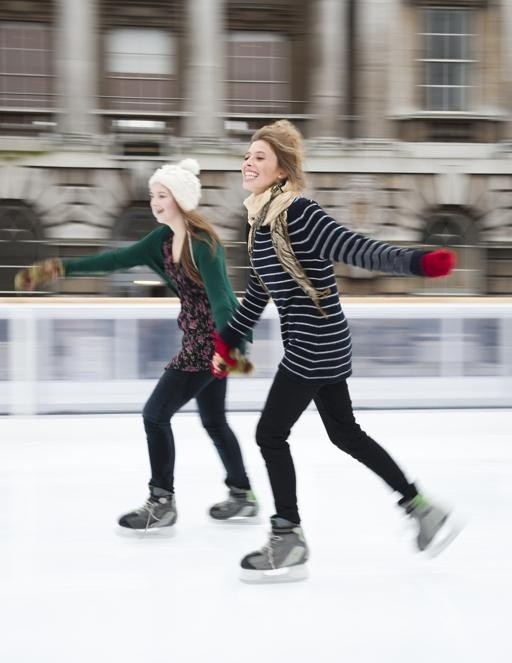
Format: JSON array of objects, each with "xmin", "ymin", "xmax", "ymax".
[
  {"xmin": 14, "ymin": 257, "xmax": 65, "ymax": 292},
  {"xmin": 417, "ymin": 250, "xmax": 457, "ymax": 277},
  {"xmin": 208, "ymin": 332, "xmax": 256, "ymax": 381}
]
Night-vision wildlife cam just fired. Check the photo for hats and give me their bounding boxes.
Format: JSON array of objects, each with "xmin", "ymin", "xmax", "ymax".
[{"xmin": 149, "ymin": 159, "xmax": 203, "ymax": 212}]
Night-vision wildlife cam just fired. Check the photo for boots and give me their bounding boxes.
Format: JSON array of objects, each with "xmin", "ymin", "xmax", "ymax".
[
  {"xmin": 241, "ymin": 511, "xmax": 308, "ymax": 570},
  {"xmin": 210, "ymin": 488, "xmax": 258, "ymax": 518},
  {"xmin": 118, "ymin": 482, "xmax": 177, "ymax": 529},
  {"xmin": 398, "ymin": 488, "xmax": 451, "ymax": 550}
]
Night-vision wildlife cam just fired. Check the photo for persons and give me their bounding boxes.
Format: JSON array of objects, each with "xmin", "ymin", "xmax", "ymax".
[
  {"xmin": 13, "ymin": 157, "xmax": 259, "ymax": 528},
  {"xmin": 210, "ymin": 119, "xmax": 457, "ymax": 569}
]
